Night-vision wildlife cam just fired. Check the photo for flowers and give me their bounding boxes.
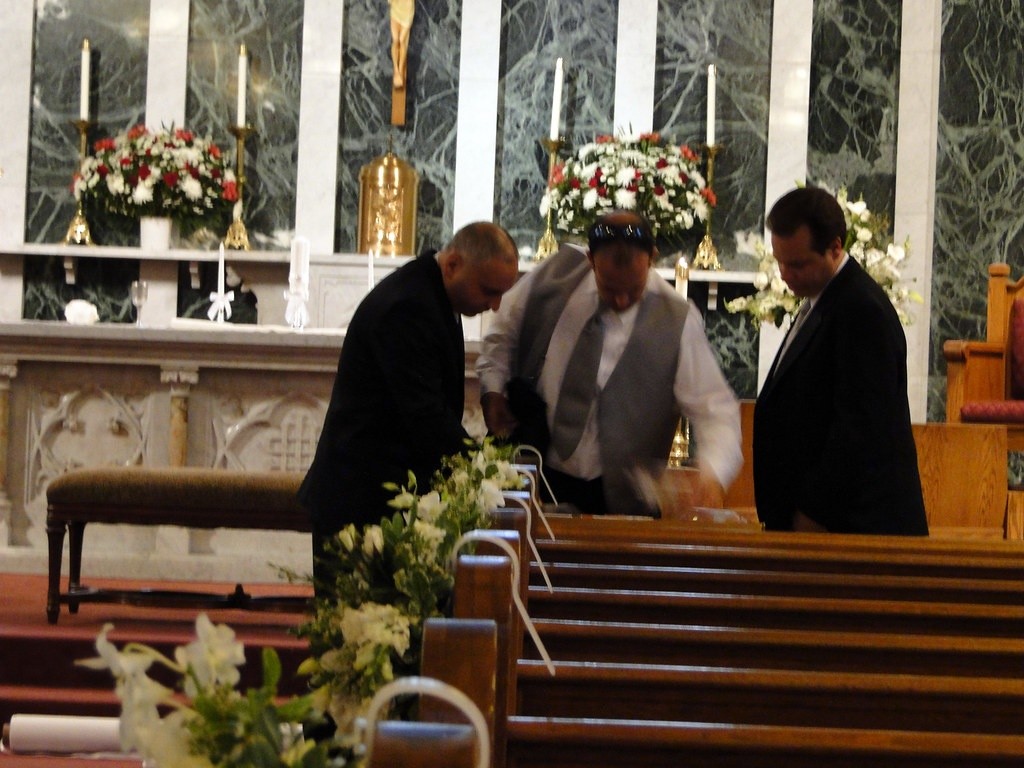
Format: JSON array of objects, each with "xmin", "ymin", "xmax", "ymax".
[
  {"xmin": 65, "ymin": 122, "xmax": 241, "ymax": 221},
  {"xmin": 96, "ymin": 433, "xmax": 531, "ymax": 768},
  {"xmin": 540, "ymin": 129, "xmax": 720, "ymax": 247},
  {"xmin": 721, "ymin": 175, "xmax": 927, "ymax": 333}
]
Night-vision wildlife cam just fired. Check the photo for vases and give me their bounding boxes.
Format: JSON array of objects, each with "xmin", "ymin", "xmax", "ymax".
[{"xmin": 139, "ymin": 211, "xmax": 182, "ymax": 249}]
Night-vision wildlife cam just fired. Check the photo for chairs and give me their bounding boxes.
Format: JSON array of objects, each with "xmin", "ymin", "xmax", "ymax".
[{"xmin": 942, "ymin": 262, "xmax": 1024, "ymax": 456}]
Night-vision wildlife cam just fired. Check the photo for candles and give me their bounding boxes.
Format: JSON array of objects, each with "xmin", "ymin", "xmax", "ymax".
[
  {"xmin": 549, "ymin": 57, "xmax": 563, "ymax": 140},
  {"xmin": 706, "ymin": 63, "xmax": 717, "ymax": 147},
  {"xmin": 236, "ymin": 43, "xmax": 248, "ymax": 128},
  {"xmin": 79, "ymin": 37, "xmax": 90, "ymax": 122}
]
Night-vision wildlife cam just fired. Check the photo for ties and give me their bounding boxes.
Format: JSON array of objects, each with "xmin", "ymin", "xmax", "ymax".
[
  {"xmin": 773, "ymin": 297, "xmax": 812, "ymax": 376},
  {"xmin": 553, "ymin": 298, "xmax": 612, "ymax": 461}
]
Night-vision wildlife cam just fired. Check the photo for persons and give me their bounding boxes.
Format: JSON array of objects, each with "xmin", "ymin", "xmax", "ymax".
[
  {"xmin": 295, "ymin": 221, "xmax": 518, "ymax": 742},
  {"xmin": 753, "ymin": 186, "xmax": 930, "ymax": 537},
  {"xmin": 477, "ymin": 211, "xmax": 745, "ymax": 525}
]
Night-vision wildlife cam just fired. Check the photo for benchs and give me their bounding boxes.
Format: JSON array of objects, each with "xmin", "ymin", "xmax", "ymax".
[{"xmin": 44, "ymin": 463, "xmax": 317, "ymax": 628}]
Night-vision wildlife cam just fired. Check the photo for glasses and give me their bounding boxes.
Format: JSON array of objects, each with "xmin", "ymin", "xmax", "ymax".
[{"xmin": 589, "ymin": 222, "xmax": 654, "ymax": 255}]
